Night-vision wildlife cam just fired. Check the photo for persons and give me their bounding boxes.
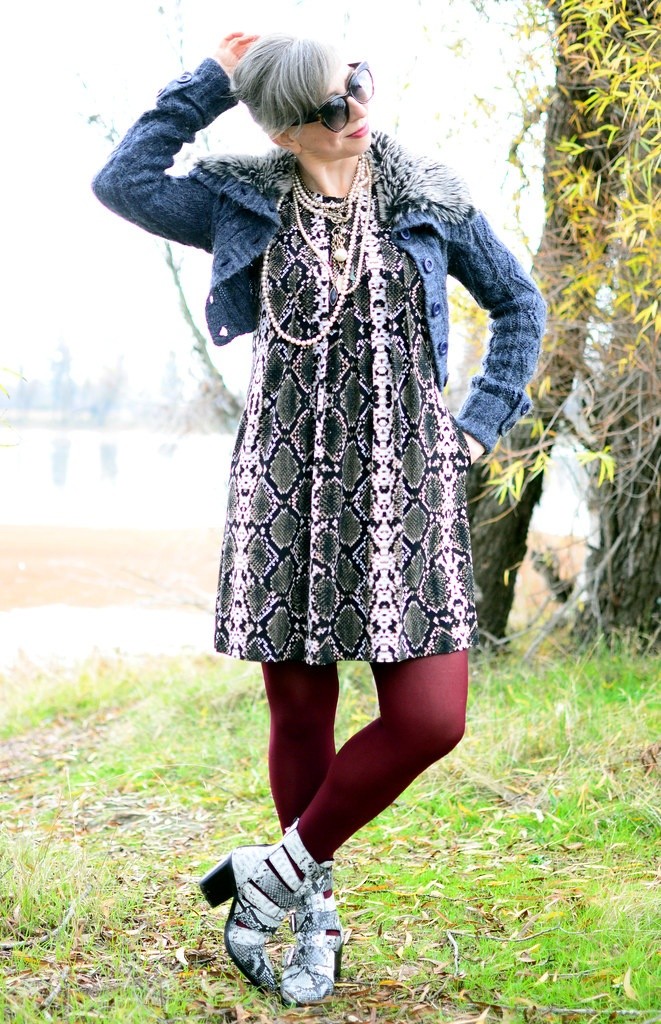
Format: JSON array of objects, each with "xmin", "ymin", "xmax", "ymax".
[{"xmin": 93, "ymin": 34, "xmax": 547, "ymax": 1002}]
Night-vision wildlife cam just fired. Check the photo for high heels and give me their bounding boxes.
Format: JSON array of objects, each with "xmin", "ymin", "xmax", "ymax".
[
  {"xmin": 199, "ymin": 816, "xmax": 335, "ymax": 995},
  {"xmin": 281, "ymin": 866, "xmax": 344, "ymax": 1006}
]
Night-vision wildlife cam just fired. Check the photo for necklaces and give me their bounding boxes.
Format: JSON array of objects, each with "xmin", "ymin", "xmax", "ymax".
[{"xmin": 261, "ymin": 154, "xmax": 372, "ymax": 347}]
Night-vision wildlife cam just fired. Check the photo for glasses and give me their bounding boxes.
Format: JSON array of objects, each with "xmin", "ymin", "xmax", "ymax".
[{"xmin": 291, "ymin": 61, "xmax": 374, "ymax": 133}]
